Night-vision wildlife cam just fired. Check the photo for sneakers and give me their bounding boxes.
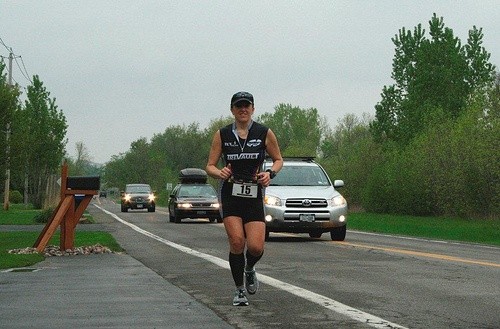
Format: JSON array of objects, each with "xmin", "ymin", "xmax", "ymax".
[
  {"xmin": 244, "ymin": 265, "xmax": 259, "ymax": 295},
  {"xmin": 232, "ymin": 287, "xmax": 249, "ymax": 306}
]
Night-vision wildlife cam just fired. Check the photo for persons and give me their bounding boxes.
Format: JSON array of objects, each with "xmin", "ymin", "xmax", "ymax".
[{"xmin": 207, "ymin": 92, "xmax": 283, "ymax": 306}]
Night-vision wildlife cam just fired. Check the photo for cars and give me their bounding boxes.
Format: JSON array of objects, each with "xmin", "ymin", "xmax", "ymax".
[
  {"xmin": 258, "ymin": 156, "xmax": 349, "ymax": 242},
  {"xmin": 99, "ymin": 191, "xmax": 106, "ymax": 198},
  {"xmin": 120, "ymin": 183, "xmax": 156, "ymax": 213},
  {"xmin": 168, "ymin": 167, "xmax": 223, "ymax": 223}
]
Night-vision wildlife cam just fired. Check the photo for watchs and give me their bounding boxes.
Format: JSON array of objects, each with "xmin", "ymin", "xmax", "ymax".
[{"xmin": 266, "ymin": 169, "xmax": 277, "ymax": 180}]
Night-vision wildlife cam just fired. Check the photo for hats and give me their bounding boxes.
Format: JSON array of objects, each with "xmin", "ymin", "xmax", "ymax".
[{"xmin": 231, "ymin": 91, "xmax": 254, "ymax": 108}]
